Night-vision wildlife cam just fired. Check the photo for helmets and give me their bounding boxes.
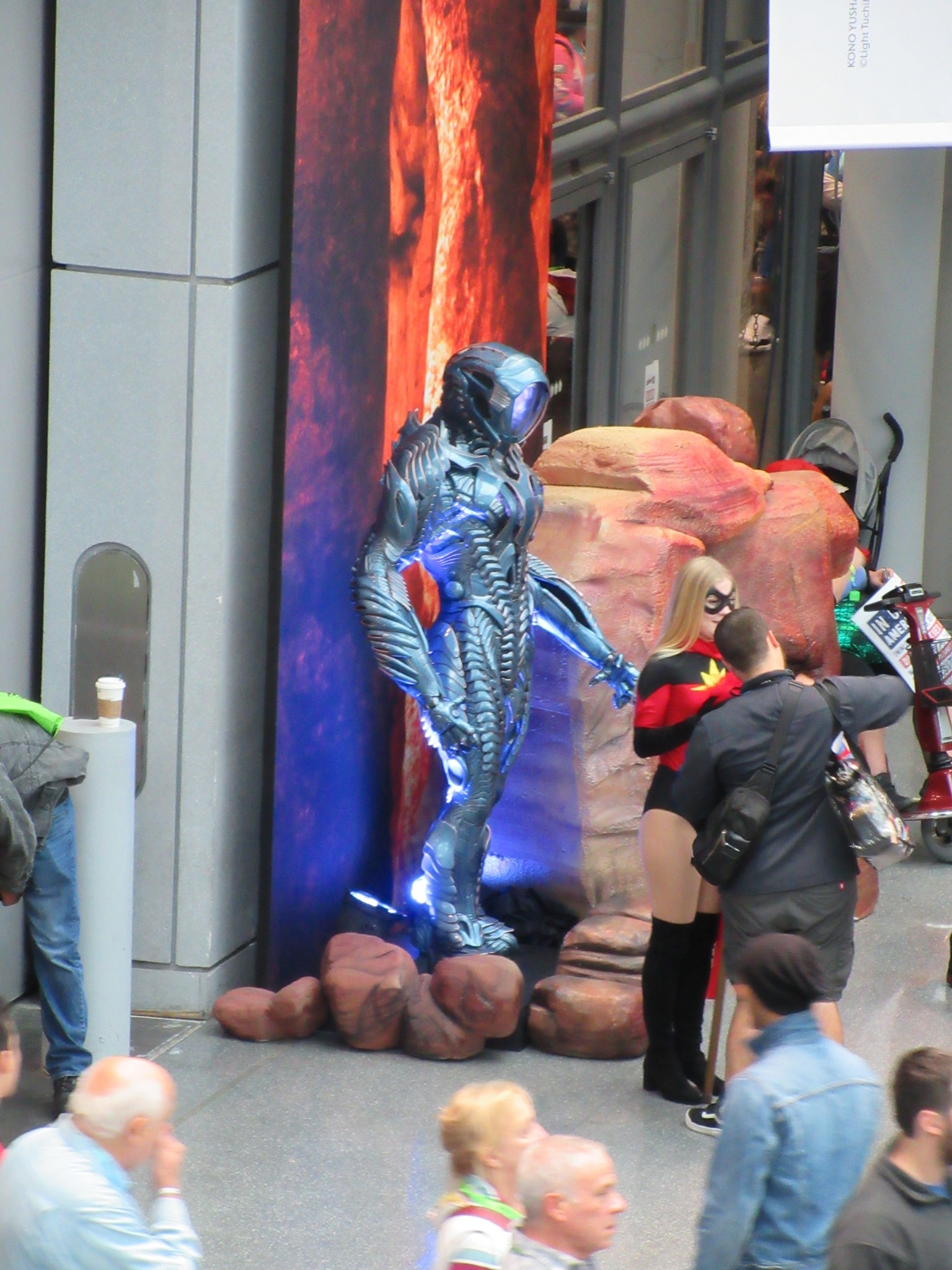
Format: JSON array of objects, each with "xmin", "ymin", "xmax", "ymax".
[{"xmin": 447, "ymin": 342, "xmax": 548, "ymax": 443}]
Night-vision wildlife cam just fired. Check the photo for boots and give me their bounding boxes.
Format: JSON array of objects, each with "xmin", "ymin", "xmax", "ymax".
[
  {"xmin": 870, "ymin": 753, "xmax": 913, "ymax": 811},
  {"xmin": 642, "ymin": 913, "xmax": 704, "ymax": 1103},
  {"xmin": 676, "ymin": 910, "xmax": 726, "ymax": 1098}
]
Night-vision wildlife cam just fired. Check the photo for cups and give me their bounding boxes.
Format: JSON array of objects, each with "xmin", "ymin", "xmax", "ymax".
[{"xmin": 95, "ymin": 677, "xmax": 125, "ymax": 728}]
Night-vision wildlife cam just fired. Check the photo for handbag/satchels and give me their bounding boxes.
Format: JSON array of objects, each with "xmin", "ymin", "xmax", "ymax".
[
  {"xmin": 691, "ymin": 681, "xmax": 803, "ymax": 885},
  {"xmin": 813, "ymin": 678, "xmax": 916, "ymax": 869}
]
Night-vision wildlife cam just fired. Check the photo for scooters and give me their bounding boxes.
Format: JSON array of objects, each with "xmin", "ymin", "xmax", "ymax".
[{"xmin": 852, "ymin": 581, "xmax": 952, "ymax": 861}]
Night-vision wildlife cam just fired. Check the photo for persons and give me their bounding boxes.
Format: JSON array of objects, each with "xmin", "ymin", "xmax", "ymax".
[
  {"xmin": 0, "ymin": 705, "xmax": 97, "ymax": 1115},
  {"xmin": 355, "ymin": 343, "xmax": 640, "ymax": 958},
  {"xmin": 544, "ymin": 236, "xmax": 581, "ymax": 343},
  {"xmin": 421, "ymin": 1080, "xmax": 549, "ymax": 1268},
  {"xmin": 548, "ymin": 6, "xmax": 588, "ymax": 120},
  {"xmin": 749, "ymin": 175, "xmax": 838, "ymax": 379},
  {"xmin": 829, "ymin": 1043, "xmax": 952, "ymax": 1270},
  {"xmin": 0, "ymin": 1006, "xmax": 23, "ymax": 1158},
  {"xmin": 827, "ymin": 545, "xmax": 917, "ymax": 811},
  {"xmin": 1, "ymin": 1056, "xmax": 206, "ymax": 1270},
  {"xmin": 494, "ymin": 1135, "xmax": 625, "ymax": 1270},
  {"xmin": 633, "ymin": 552, "xmax": 752, "ymax": 1106},
  {"xmin": 691, "ymin": 930, "xmax": 882, "ymax": 1270},
  {"xmin": 668, "ymin": 604, "xmax": 916, "ymax": 1136}
]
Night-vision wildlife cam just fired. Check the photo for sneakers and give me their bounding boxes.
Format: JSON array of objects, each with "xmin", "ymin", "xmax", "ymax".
[
  {"xmin": 52, "ymin": 1075, "xmax": 77, "ymax": 1118},
  {"xmin": 684, "ymin": 1099, "xmax": 722, "ymax": 1140}
]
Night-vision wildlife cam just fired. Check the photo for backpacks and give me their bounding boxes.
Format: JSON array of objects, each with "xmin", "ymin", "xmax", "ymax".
[{"xmin": 0, "ymin": 690, "xmax": 64, "ymax": 781}]
